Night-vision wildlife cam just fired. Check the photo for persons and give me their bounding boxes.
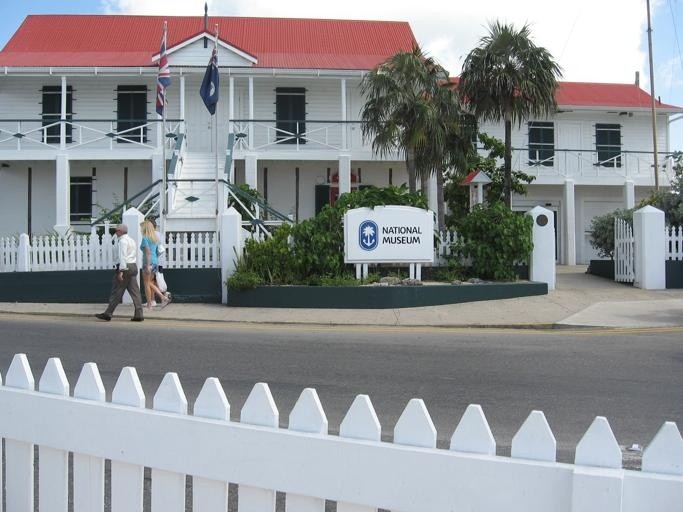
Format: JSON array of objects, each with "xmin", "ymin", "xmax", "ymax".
[{"xmin": 94, "ymin": 223, "xmax": 145, "ymax": 322}]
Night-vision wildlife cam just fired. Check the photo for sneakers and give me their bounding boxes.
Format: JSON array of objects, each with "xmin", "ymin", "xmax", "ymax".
[{"xmin": 141, "ymin": 292, "xmax": 171, "ymax": 312}]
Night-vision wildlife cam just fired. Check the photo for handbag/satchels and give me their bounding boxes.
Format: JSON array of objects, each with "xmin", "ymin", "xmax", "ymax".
[
  {"xmin": 156, "ymin": 242, "xmax": 166, "ymax": 257},
  {"xmin": 155, "ymin": 271, "xmax": 168, "ymax": 293}
]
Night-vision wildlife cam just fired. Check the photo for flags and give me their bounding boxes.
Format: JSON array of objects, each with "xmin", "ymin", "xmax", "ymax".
[
  {"xmin": 155, "ymin": 31, "xmax": 172, "ymax": 116},
  {"xmin": 199, "ymin": 36, "xmax": 220, "ymax": 116}
]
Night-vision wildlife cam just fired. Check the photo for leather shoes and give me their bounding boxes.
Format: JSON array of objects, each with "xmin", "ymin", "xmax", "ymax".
[
  {"xmin": 131, "ymin": 317, "xmax": 144, "ymax": 322},
  {"xmin": 95, "ymin": 313, "xmax": 112, "ymax": 321}
]
